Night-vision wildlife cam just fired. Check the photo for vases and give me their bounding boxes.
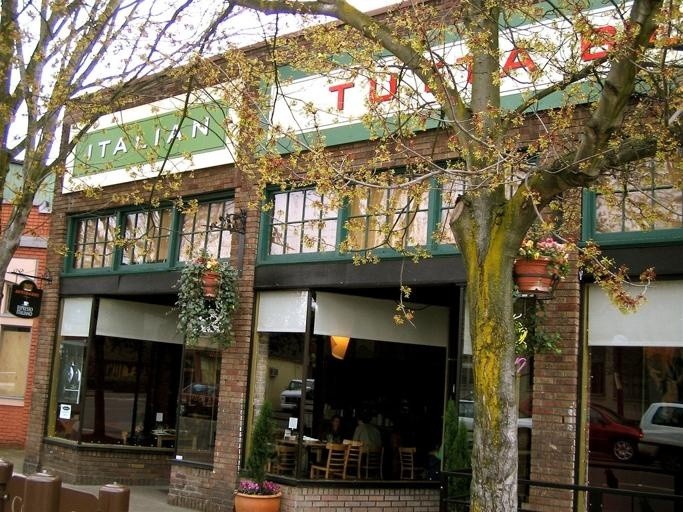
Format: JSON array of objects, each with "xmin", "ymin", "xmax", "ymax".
[
  {"xmin": 514, "ymin": 258, "xmax": 562, "ymax": 293},
  {"xmin": 234, "ymin": 489, "xmax": 282, "ymax": 512}
]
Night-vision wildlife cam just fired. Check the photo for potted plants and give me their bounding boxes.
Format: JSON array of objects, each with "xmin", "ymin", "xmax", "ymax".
[{"xmin": 166, "ymin": 251, "xmax": 244, "ymax": 347}]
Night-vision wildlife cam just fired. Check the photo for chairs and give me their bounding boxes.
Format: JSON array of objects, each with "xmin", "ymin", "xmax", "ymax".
[{"xmin": 268, "ymin": 422, "xmax": 438, "ymax": 481}]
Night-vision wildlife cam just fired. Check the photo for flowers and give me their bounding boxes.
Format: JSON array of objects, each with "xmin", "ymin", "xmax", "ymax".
[
  {"xmin": 518, "ymin": 238, "xmax": 570, "ymax": 260},
  {"xmin": 236, "ymin": 476, "xmax": 279, "ymax": 496}
]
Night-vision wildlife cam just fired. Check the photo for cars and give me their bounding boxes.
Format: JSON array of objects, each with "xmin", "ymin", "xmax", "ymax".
[
  {"xmin": 179, "ymin": 382, "xmax": 218, "ymax": 419},
  {"xmin": 588, "ymin": 401, "xmax": 642, "ymax": 463},
  {"xmin": 456, "ymin": 399, "xmax": 533, "ymax": 449}
]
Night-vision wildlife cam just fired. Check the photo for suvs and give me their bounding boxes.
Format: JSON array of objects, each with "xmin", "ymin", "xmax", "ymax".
[
  {"xmin": 280, "ymin": 378, "xmax": 314, "ymax": 410},
  {"xmin": 638, "ymin": 399, "xmax": 682, "ymax": 469}
]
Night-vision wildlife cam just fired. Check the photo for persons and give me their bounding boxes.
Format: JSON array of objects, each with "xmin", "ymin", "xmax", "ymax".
[
  {"xmin": 351, "ymin": 415, "xmax": 380, "ymax": 473},
  {"xmin": 319, "ymin": 413, "xmax": 343, "ymax": 444}
]
